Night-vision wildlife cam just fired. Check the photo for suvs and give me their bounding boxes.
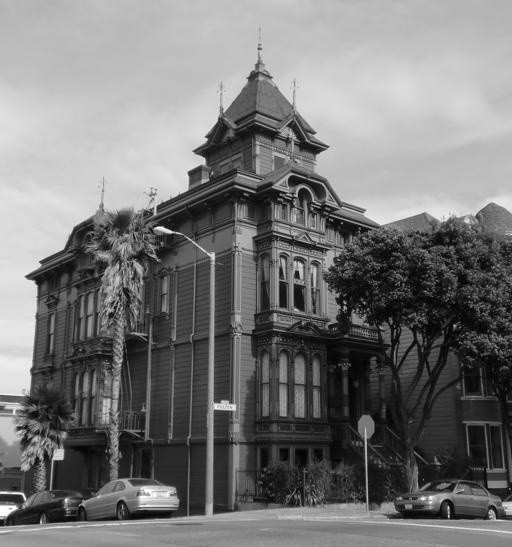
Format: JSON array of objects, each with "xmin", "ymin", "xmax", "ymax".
[{"xmin": 0, "ymin": 490, "xmax": 27, "ymax": 527}]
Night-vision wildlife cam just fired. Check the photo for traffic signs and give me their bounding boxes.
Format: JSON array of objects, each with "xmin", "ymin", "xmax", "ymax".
[{"xmin": 215, "ymin": 399, "xmax": 238, "ymax": 412}]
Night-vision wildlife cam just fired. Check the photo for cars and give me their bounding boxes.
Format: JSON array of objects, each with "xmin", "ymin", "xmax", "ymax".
[
  {"xmin": 4, "ymin": 490, "xmax": 88, "ymax": 526},
  {"xmin": 395, "ymin": 477, "xmax": 506, "ymax": 522},
  {"xmin": 77, "ymin": 475, "xmax": 181, "ymax": 521},
  {"xmin": 502, "ymin": 493, "xmax": 512, "ymax": 519}
]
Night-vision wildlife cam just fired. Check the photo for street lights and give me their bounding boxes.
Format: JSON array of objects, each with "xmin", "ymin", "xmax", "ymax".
[{"xmin": 153, "ymin": 224, "xmax": 214, "ymax": 517}]
